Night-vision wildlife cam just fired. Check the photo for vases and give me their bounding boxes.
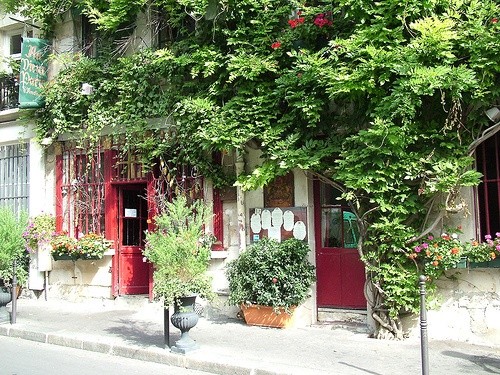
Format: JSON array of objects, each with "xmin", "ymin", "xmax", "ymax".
[
  {"xmin": 51, "ymin": 252, "xmax": 99, "ymax": 262},
  {"xmin": 38, "ymin": 240, "xmax": 53, "ymax": 272},
  {"xmin": 470, "ymin": 256, "xmax": 500, "ymax": 268}
]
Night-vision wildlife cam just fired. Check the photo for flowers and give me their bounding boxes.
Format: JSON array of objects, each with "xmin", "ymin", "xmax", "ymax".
[
  {"xmin": 51, "ymin": 231, "xmax": 111, "ymax": 258},
  {"xmin": 466, "ymin": 232, "xmax": 500, "ymax": 264},
  {"xmin": 22, "ymin": 210, "xmax": 60, "ymax": 254}
]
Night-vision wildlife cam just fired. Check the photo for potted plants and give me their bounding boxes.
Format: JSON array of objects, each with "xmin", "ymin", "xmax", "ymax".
[
  {"xmin": 222, "ymin": 235, "xmax": 319, "ymax": 328},
  {"xmin": 0, "ymin": 205, "xmax": 28, "ymax": 323},
  {"xmin": 139, "ymin": 195, "xmax": 217, "ymax": 356}
]
